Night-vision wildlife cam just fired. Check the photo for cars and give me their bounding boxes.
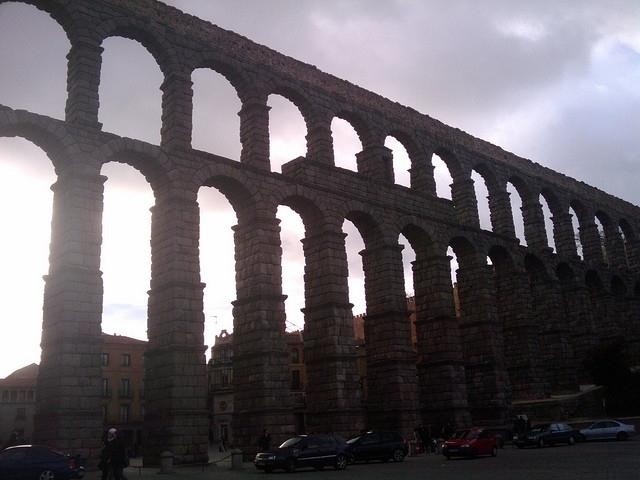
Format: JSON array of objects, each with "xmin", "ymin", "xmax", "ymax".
[
  {"xmin": 346, "ymin": 431, "xmax": 408, "ymax": 464},
  {"xmin": 254, "ymin": 435, "xmax": 352, "ymax": 473},
  {"xmin": 576, "ymin": 420, "xmax": 636, "ymax": 442},
  {"xmin": 442, "ymin": 426, "xmax": 506, "ymax": 459},
  {"xmin": 0, "ymin": 445, "xmax": 85, "ymax": 480},
  {"xmin": 512, "ymin": 424, "xmax": 580, "ymax": 449}
]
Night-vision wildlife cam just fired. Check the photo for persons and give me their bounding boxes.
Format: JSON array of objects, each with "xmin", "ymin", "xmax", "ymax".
[
  {"xmin": 417, "ymin": 421, "xmax": 456, "ymax": 452},
  {"xmin": 100, "ymin": 426, "xmax": 127, "ymax": 480},
  {"xmin": 260, "ymin": 429, "xmax": 273, "ymax": 450}
]
